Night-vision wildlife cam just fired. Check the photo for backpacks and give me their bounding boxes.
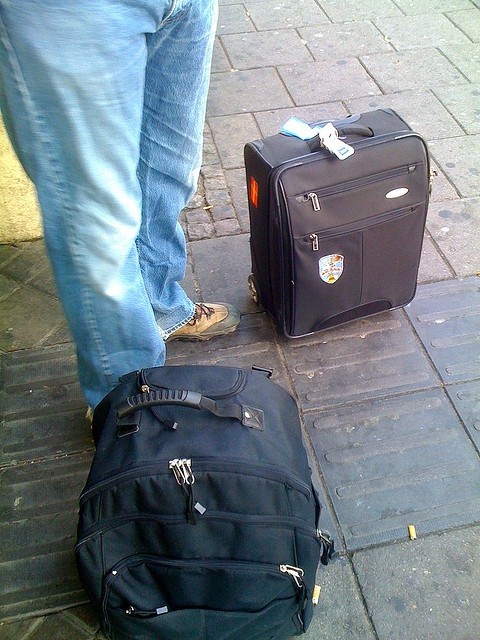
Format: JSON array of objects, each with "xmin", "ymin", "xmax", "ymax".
[{"xmin": 73, "ymin": 365, "xmax": 335, "ymax": 640}]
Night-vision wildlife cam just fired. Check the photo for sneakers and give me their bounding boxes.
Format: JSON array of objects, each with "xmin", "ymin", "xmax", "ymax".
[{"xmin": 165, "ymin": 302, "xmax": 242, "ymax": 343}]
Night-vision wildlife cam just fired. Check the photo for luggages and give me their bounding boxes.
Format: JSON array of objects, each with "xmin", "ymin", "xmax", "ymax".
[{"xmin": 244, "ymin": 108, "xmax": 432, "ymax": 339}]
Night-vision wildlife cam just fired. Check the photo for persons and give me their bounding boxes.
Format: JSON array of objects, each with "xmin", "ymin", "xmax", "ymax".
[{"xmin": 1, "ymin": 2, "xmax": 244, "ymax": 427}]
